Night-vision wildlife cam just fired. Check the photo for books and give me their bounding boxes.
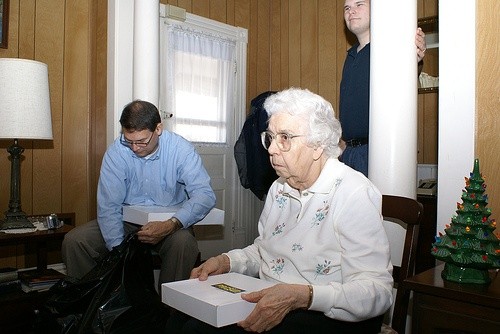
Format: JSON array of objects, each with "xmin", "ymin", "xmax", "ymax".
[{"xmin": 19, "ymin": 269, "xmax": 66, "ymax": 293}]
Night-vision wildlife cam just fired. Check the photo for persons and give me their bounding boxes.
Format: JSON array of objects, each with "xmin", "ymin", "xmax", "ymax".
[
  {"xmin": 189, "ymin": 87, "xmax": 394, "ymax": 334},
  {"xmin": 61, "ymin": 99, "xmax": 216, "ymax": 334},
  {"xmin": 340, "ymin": 0, "xmax": 426, "ymax": 180}
]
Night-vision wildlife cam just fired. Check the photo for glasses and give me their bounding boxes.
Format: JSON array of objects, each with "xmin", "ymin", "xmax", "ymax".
[
  {"xmin": 120, "ymin": 132, "xmax": 154, "ymax": 147},
  {"xmin": 260, "ymin": 131, "xmax": 300, "ymax": 152}
]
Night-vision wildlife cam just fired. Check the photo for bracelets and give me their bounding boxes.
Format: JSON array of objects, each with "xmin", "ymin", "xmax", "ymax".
[
  {"xmin": 304, "ymin": 285, "xmax": 313, "ymax": 309},
  {"xmin": 171, "ymin": 218, "xmax": 179, "ymax": 229}
]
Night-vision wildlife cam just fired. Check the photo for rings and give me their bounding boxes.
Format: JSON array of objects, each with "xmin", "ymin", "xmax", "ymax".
[{"xmin": 422, "ymin": 48, "xmax": 426, "ymax": 52}]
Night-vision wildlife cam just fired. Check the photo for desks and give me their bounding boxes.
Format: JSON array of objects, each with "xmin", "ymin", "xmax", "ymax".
[
  {"xmin": 415, "ymin": 195, "xmax": 437, "ymax": 271},
  {"xmin": 402, "ymin": 262, "xmax": 500, "ymax": 334}
]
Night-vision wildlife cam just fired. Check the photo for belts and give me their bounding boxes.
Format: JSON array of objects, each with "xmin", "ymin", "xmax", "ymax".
[{"xmin": 346, "ymin": 138, "xmax": 368, "ymax": 147}]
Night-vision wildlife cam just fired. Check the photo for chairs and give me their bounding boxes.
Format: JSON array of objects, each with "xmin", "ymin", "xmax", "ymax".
[{"xmin": 382, "ymin": 195, "xmax": 423, "ymax": 334}]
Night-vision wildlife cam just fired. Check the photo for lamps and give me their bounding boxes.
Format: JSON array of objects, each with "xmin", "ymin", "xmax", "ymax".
[{"xmin": 0, "ymin": 57, "xmax": 54, "ymax": 228}]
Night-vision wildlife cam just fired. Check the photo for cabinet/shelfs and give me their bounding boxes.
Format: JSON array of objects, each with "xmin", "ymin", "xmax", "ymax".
[{"xmin": 0, "ymin": 224, "xmax": 75, "ymax": 334}]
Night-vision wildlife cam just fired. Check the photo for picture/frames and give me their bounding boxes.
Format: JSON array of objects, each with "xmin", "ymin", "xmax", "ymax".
[{"xmin": 0, "ymin": 0, "xmax": 10, "ymax": 49}]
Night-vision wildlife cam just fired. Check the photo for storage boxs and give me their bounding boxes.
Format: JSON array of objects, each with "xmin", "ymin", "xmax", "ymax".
[
  {"xmin": 122, "ymin": 205, "xmax": 225, "ymax": 226},
  {"xmin": 160, "ymin": 271, "xmax": 275, "ymax": 328}
]
